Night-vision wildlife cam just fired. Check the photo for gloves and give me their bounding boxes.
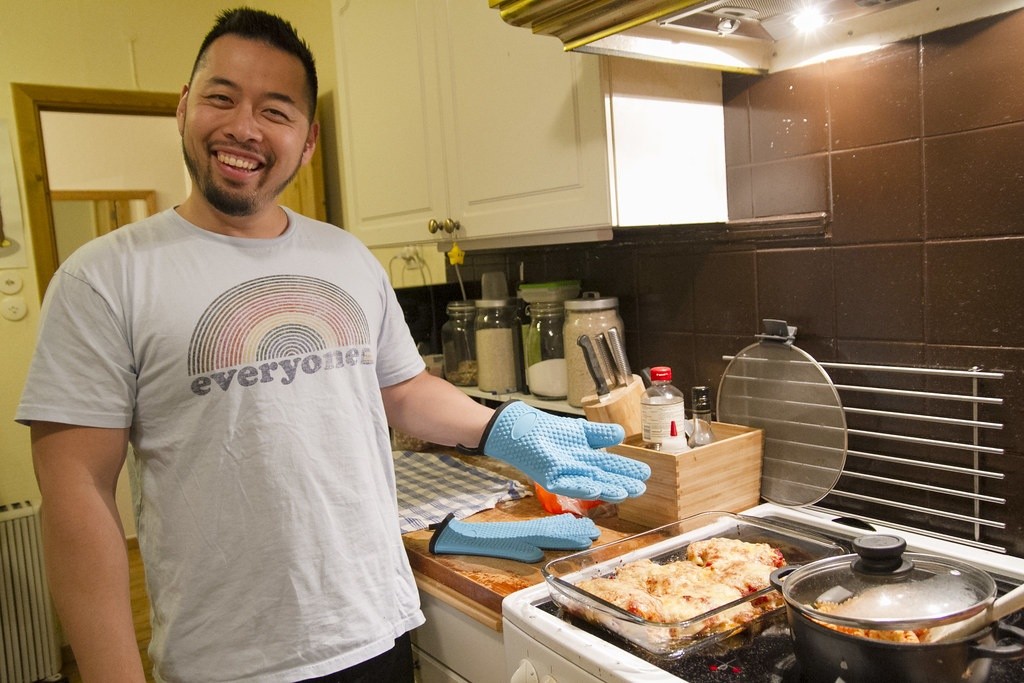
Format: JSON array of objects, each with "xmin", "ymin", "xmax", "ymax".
[
  {"xmin": 427, "ymin": 512, "xmax": 601, "ymax": 564},
  {"xmin": 457, "ymin": 399, "xmax": 652, "ymax": 505}
]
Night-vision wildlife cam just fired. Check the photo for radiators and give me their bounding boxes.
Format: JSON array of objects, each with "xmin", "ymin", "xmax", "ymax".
[{"xmin": 0, "ymin": 499, "xmax": 64, "ymax": 682}]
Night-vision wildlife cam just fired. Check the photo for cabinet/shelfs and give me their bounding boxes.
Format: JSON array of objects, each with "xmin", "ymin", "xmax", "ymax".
[
  {"xmin": 409, "ymin": 587, "xmax": 507, "ymax": 683},
  {"xmin": 329, "ymin": 2, "xmax": 733, "ymax": 251}
]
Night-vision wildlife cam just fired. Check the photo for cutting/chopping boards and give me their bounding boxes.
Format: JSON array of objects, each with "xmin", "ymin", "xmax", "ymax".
[{"xmin": 400, "ymin": 453, "xmax": 672, "ymax": 614}]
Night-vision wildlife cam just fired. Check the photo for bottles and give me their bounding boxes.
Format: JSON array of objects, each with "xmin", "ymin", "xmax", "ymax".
[
  {"xmin": 640, "ymin": 367, "xmax": 685, "ymax": 454},
  {"xmin": 688, "ymin": 387, "xmax": 719, "ymax": 449}
]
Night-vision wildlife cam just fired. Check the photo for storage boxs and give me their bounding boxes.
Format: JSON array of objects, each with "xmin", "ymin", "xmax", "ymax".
[{"xmin": 604, "ymin": 419, "xmax": 766, "ymax": 536}]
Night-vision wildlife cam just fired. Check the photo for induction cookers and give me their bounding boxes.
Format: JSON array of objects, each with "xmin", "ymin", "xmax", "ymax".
[{"xmin": 503, "ymin": 502, "xmax": 1024, "ymax": 683}]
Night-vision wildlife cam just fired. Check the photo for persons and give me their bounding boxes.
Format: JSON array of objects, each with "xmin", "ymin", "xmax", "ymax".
[{"xmin": 13, "ymin": 6, "xmax": 651, "ymax": 683}]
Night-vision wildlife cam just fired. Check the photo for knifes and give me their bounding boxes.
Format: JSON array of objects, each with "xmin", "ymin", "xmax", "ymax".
[{"xmin": 576, "ymin": 327, "xmax": 646, "ymax": 437}]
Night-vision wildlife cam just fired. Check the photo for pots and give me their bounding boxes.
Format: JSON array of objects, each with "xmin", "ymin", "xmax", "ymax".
[{"xmin": 770, "ymin": 534, "xmax": 1024, "ymax": 683}]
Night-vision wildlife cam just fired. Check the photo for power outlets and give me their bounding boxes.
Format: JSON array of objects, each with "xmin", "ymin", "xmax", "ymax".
[{"xmin": 403, "ymin": 244, "xmax": 426, "ymax": 271}]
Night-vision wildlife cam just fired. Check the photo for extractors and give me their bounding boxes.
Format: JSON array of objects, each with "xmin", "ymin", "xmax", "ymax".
[{"xmin": 490, "ymin": 0, "xmax": 1024, "ymax": 76}]
